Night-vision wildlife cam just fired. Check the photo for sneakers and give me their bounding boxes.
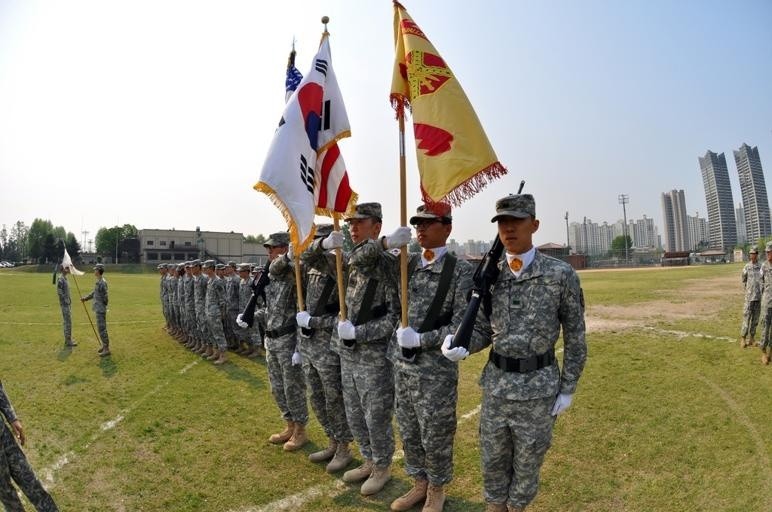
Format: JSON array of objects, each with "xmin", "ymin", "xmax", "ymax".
[
  {"xmin": 740, "ymin": 336, "xmax": 748, "ymax": 348},
  {"xmin": 63, "ymin": 338, "xmax": 78, "ymax": 347},
  {"xmin": 762, "ymin": 350, "xmax": 772, "ymax": 366},
  {"xmin": 161, "ymin": 324, "xmax": 192, "ymax": 347},
  {"xmin": 749, "ymin": 334, "xmax": 755, "ymax": 345}
]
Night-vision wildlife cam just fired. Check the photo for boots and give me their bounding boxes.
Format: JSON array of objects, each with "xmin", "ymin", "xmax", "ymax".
[
  {"xmin": 195, "ymin": 343, "xmax": 213, "ymax": 358},
  {"xmin": 421, "ymin": 482, "xmax": 446, "ymax": 512},
  {"xmin": 483, "ymin": 502, "xmax": 527, "ymax": 512},
  {"xmin": 282, "ymin": 422, "xmax": 308, "ymax": 452},
  {"xmin": 97, "ymin": 346, "xmax": 111, "ymax": 357},
  {"xmin": 225, "ymin": 336, "xmax": 262, "ymax": 359},
  {"xmin": 326, "ymin": 443, "xmax": 353, "ymax": 474},
  {"xmin": 342, "ymin": 459, "xmax": 375, "ymax": 483},
  {"xmin": 186, "ymin": 339, "xmax": 201, "ymax": 352},
  {"xmin": 206, "ymin": 348, "xmax": 219, "ymax": 361},
  {"xmin": 268, "ymin": 420, "xmax": 295, "ymax": 444},
  {"xmin": 214, "ymin": 352, "xmax": 225, "ymax": 366},
  {"xmin": 308, "ymin": 438, "xmax": 337, "ymax": 464},
  {"xmin": 389, "ymin": 478, "xmax": 428, "ymax": 512},
  {"xmin": 360, "ymin": 464, "xmax": 393, "ymax": 497}
]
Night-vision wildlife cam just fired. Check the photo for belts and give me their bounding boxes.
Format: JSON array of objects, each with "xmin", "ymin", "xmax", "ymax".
[
  {"xmin": 264, "ymin": 323, "xmax": 296, "ymax": 339},
  {"xmin": 488, "ymin": 347, "xmax": 556, "ymax": 374}
]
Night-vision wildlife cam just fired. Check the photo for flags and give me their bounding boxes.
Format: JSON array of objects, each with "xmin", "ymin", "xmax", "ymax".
[
  {"xmin": 257, "ymin": 34, "xmax": 352, "ymax": 248},
  {"xmin": 387, "ymin": 0, "xmax": 500, "ymax": 204},
  {"xmin": 61, "ymin": 247, "xmax": 84, "ymax": 276},
  {"xmin": 283, "ymin": 47, "xmax": 357, "ymax": 213}
]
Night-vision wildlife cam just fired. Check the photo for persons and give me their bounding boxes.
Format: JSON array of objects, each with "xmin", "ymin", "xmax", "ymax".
[
  {"xmin": 757, "ymin": 240, "xmax": 771, "ymax": 365},
  {"xmin": 740, "ymin": 245, "xmax": 763, "ymax": 349},
  {"xmin": 349, "ymin": 200, "xmax": 473, "ymax": 512},
  {"xmin": 156, "ymin": 258, "xmax": 265, "ymax": 366},
  {"xmin": 80, "ymin": 263, "xmax": 112, "ymax": 356},
  {"xmin": 268, "ymin": 223, "xmax": 355, "ymax": 474},
  {"xmin": 1, "ymin": 381, "xmax": 60, "ymax": 512},
  {"xmin": 57, "ymin": 263, "xmax": 78, "ymax": 346},
  {"xmin": 440, "ymin": 193, "xmax": 588, "ymax": 512},
  {"xmin": 298, "ymin": 202, "xmax": 398, "ymax": 496},
  {"xmin": 235, "ymin": 231, "xmax": 311, "ymax": 451}
]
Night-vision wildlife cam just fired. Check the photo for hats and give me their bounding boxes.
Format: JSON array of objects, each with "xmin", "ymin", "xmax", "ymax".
[
  {"xmin": 343, "ymin": 202, "xmax": 383, "ymax": 222},
  {"xmin": 409, "ymin": 202, "xmax": 453, "ymax": 225},
  {"xmin": 250, "ymin": 262, "xmax": 266, "ymax": 274},
  {"xmin": 224, "ymin": 260, "xmax": 236, "ymax": 267},
  {"xmin": 313, "ymin": 223, "xmax": 335, "ymax": 237},
  {"xmin": 157, "ymin": 259, "xmax": 216, "ymax": 272},
  {"xmin": 215, "ymin": 264, "xmax": 226, "ymax": 270},
  {"xmin": 93, "ymin": 264, "xmax": 104, "ymax": 271},
  {"xmin": 749, "ymin": 248, "xmax": 758, "ymax": 254},
  {"xmin": 235, "ymin": 264, "xmax": 250, "ymax": 272},
  {"xmin": 262, "ymin": 231, "xmax": 290, "ymax": 248},
  {"xmin": 490, "ymin": 193, "xmax": 536, "ymax": 224},
  {"xmin": 764, "ymin": 244, "xmax": 772, "ymax": 252}
]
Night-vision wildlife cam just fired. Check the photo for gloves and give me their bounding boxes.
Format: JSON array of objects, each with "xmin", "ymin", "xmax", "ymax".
[
  {"xmin": 440, "ymin": 334, "xmax": 470, "ymax": 363},
  {"xmin": 291, "ymin": 352, "xmax": 303, "ymax": 367},
  {"xmin": 285, "ymin": 241, "xmax": 296, "ymax": 262},
  {"xmin": 551, "ymin": 393, "xmax": 573, "ymax": 417},
  {"xmin": 235, "ymin": 313, "xmax": 249, "ymax": 330},
  {"xmin": 395, "ymin": 326, "xmax": 422, "ymax": 350},
  {"xmin": 322, "ymin": 229, "xmax": 344, "ymax": 249},
  {"xmin": 385, "ymin": 226, "xmax": 412, "ymax": 249},
  {"xmin": 337, "ymin": 319, "xmax": 356, "ymax": 341},
  {"xmin": 295, "ymin": 310, "xmax": 312, "ymax": 330}
]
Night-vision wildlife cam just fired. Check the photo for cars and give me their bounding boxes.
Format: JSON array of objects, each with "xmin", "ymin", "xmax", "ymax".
[{"xmin": 0, "ymin": 260, "xmax": 17, "ymax": 268}]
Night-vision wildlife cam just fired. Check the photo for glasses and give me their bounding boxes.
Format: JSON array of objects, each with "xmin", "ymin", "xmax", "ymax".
[{"xmin": 412, "ymin": 220, "xmax": 448, "ymax": 229}]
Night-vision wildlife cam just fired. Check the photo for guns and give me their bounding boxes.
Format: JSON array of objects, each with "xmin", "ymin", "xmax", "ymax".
[
  {"xmin": 241, "ymin": 225, "xmax": 290, "ymax": 328},
  {"xmin": 449, "ymin": 180, "xmax": 526, "ymax": 360}
]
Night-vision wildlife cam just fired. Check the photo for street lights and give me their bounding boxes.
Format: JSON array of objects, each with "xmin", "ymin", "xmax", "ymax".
[
  {"xmin": 618, "ymin": 192, "xmax": 633, "ymax": 267},
  {"xmin": 739, "ymin": 170, "xmax": 756, "ymax": 249}
]
